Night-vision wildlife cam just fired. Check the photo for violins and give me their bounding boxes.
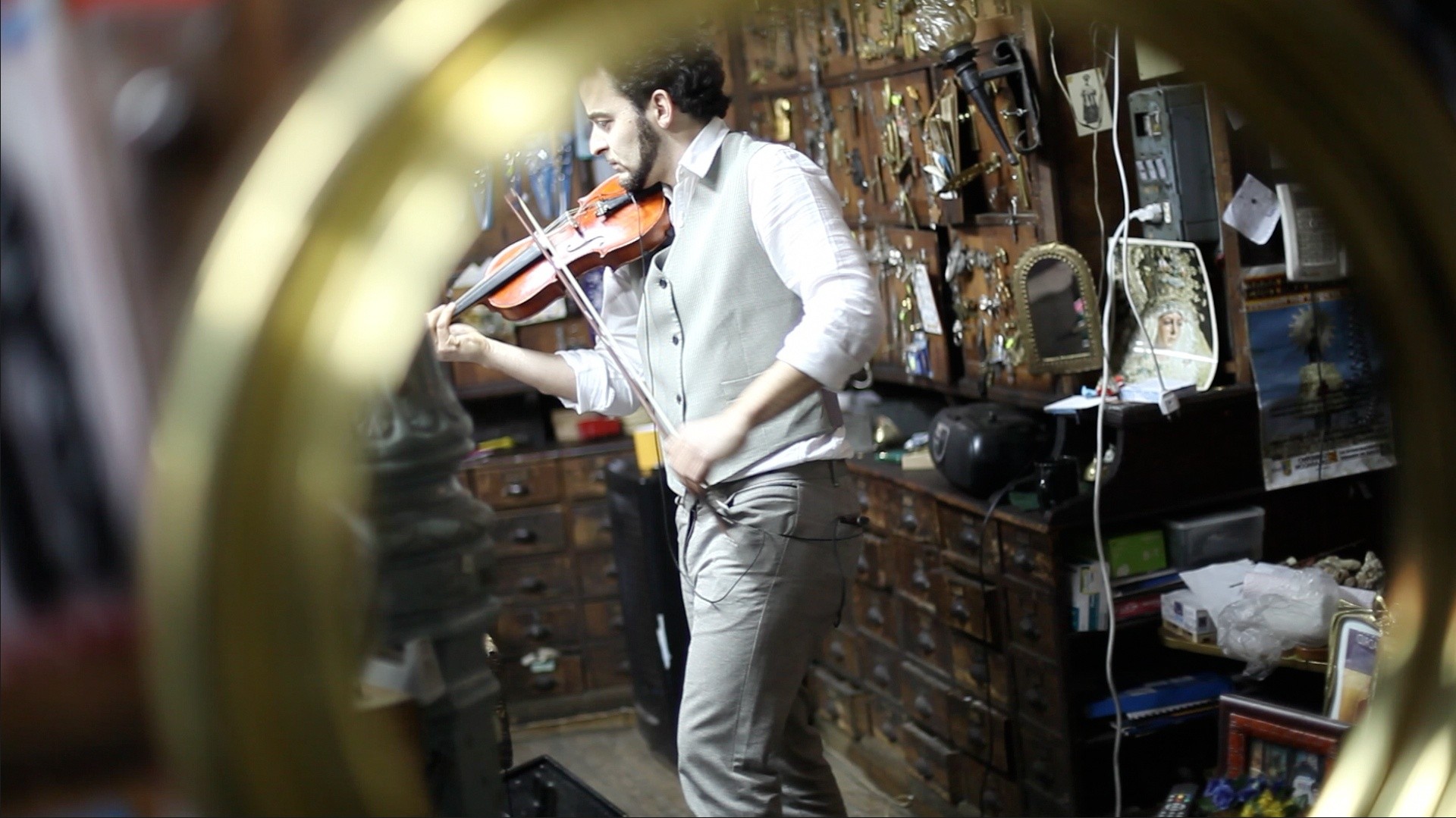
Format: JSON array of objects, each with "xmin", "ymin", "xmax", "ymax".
[{"xmin": 450, "ymin": 173, "xmax": 673, "ymax": 322}]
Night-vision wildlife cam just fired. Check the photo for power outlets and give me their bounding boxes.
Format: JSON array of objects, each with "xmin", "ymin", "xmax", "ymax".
[{"xmin": 1161, "ymin": 201, "xmax": 1172, "ymax": 223}]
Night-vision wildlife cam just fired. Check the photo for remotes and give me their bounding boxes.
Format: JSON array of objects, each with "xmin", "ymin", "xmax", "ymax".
[{"xmin": 1158, "ymin": 783, "xmax": 1200, "ymax": 817}]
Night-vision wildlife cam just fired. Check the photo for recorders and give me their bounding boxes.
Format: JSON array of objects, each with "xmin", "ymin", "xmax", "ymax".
[{"xmin": 927, "ymin": 401, "xmax": 1052, "ymax": 498}]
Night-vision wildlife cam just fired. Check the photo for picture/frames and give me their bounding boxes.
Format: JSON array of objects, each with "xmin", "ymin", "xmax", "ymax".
[{"xmin": 1217, "ymin": 692, "xmax": 1350, "ymax": 808}]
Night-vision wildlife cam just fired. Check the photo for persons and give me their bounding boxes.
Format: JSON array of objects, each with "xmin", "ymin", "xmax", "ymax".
[
  {"xmin": 423, "ymin": 26, "xmax": 881, "ymax": 818},
  {"xmin": 1122, "ymin": 254, "xmax": 1206, "ymax": 380}
]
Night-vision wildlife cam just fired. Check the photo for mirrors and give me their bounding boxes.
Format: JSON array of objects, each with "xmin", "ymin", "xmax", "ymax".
[
  {"xmin": 141, "ymin": 0, "xmax": 1456, "ymax": 817},
  {"xmin": 1010, "ymin": 244, "xmax": 1104, "ymax": 376}
]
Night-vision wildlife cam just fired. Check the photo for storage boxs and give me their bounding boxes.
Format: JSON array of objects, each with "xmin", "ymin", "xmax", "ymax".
[{"xmin": 1169, "ymin": 507, "xmax": 1266, "ymax": 572}]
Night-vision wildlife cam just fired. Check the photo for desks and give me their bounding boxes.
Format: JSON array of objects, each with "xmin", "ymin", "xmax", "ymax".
[{"xmin": 1159, "ymin": 542, "xmax": 1356, "ymax": 673}]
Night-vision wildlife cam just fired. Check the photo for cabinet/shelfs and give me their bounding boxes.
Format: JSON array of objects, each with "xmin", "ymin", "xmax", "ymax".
[
  {"xmin": 722, "ymin": 1, "xmax": 1251, "ymax": 818},
  {"xmin": 466, "ymin": 447, "xmax": 634, "ymax": 724}
]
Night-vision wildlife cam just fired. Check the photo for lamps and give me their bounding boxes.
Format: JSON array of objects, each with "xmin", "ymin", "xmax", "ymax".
[{"xmin": 912, "ymin": 0, "xmax": 1042, "ymax": 166}]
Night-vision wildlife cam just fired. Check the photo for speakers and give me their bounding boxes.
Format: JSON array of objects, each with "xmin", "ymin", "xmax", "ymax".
[{"xmin": 601, "ymin": 460, "xmax": 693, "ymax": 763}]
